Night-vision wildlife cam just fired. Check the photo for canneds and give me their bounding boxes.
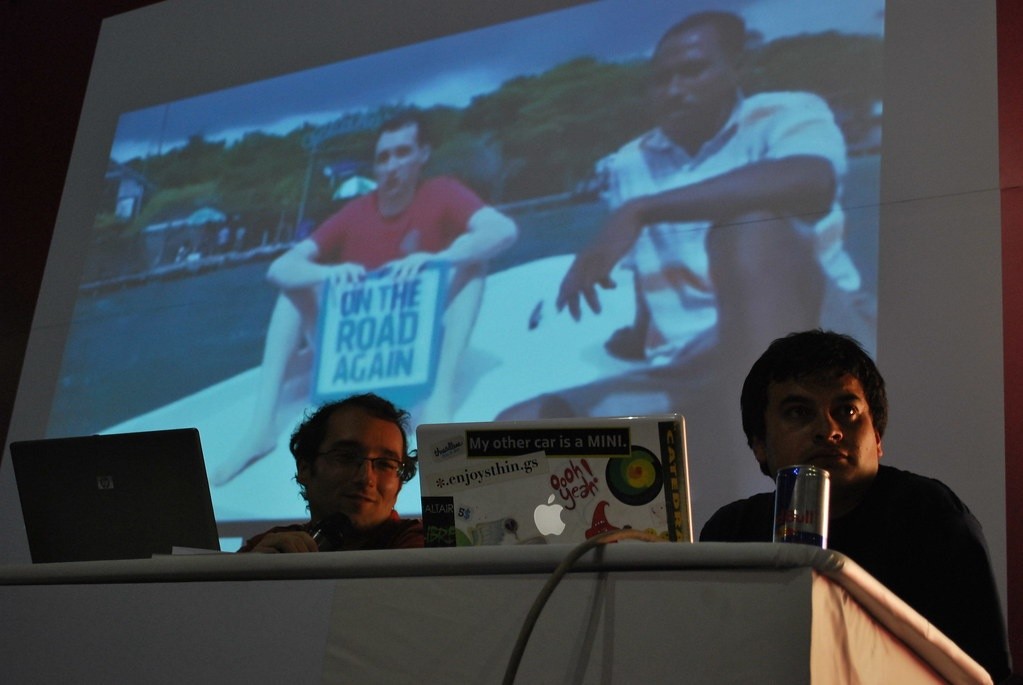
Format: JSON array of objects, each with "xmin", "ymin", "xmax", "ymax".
[{"xmin": 772, "ymin": 464, "xmax": 831, "ymax": 551}]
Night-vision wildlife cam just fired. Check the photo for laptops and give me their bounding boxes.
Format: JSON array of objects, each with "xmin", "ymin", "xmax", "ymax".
[
  {"xmin": 417, "ymin": 414, "xmax": 693, "ymax": 549},
  {"xmin": 11, "ymin": 427, "xmax": 221, "ymax": 564}
]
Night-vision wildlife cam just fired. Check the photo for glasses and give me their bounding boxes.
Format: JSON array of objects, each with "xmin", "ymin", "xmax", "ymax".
[{"xmin": 306, "ymin": 454, "xmax": 407, "ymax": 479}]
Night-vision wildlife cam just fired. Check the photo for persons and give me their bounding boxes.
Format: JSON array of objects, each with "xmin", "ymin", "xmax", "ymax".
[
  {"xmin": 697, "ymin": 329, "xmax": 1012, "ymax": 685},
  {"xmin": 209, "ymin": 108, "xmax": 519, "ymax": 485},
  {"xmin": 489, "ymin": 10, "xmax": 861, "ymax": 488},
  {"xmin": 232, "ymin": 394, "xmax": 421, "ymax": 555}
]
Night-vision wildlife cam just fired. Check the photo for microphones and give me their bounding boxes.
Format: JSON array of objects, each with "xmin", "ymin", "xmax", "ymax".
[{"xmin": 310, "ymin": 513, "xmax": 353, "ymax": 552}]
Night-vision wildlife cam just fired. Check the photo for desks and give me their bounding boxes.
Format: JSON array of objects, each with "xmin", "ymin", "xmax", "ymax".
[{"xmin": 0, "ymin": 542, "xmax": 997, "ymax": 685}]
